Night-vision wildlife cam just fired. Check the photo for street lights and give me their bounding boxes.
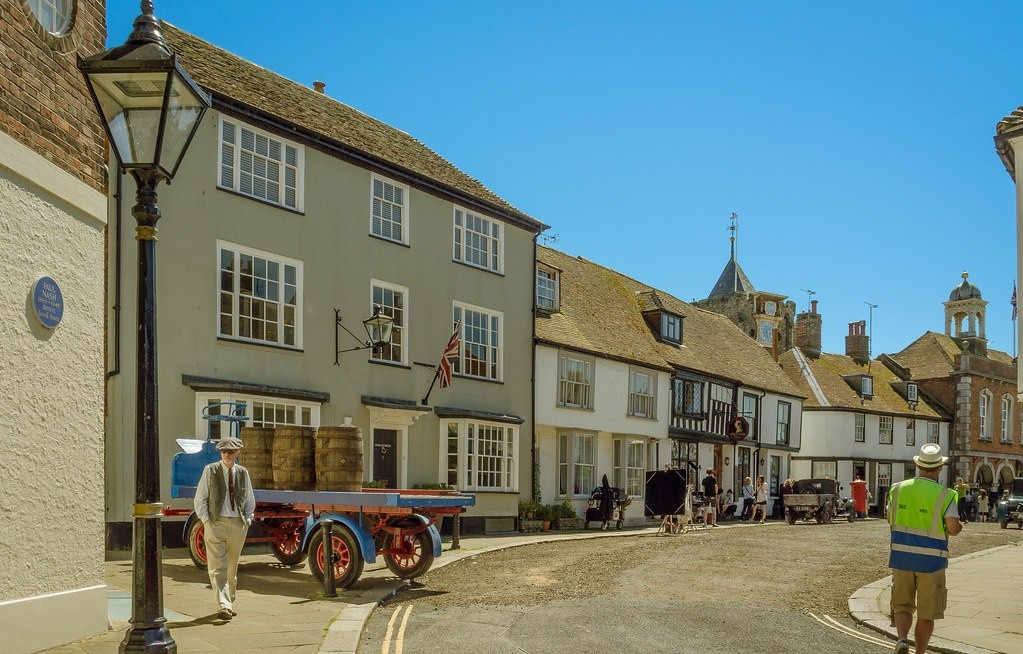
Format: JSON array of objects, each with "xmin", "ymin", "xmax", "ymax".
[{"xmin": 74, "ymin": 0, "xmax": 213, "ymax": 653}]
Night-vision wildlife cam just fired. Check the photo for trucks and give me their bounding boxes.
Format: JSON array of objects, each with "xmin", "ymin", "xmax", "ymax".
[{"xmin": 784, "ymin": 478, "xmax": 844, "ymax": 524}]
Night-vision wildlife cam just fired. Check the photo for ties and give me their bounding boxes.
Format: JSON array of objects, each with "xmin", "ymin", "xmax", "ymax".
[{"xmin": 229, "ymin": 468, "xmax": 235, "ymax": 512}]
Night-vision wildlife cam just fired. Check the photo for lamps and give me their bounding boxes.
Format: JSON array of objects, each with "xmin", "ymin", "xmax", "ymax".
[
  {"xmin": 725, "ymin": 457, "xmax": 730, "ymax": 466},
  {"xmin": 760, "ymin": 458, "xmax": 765, "ymax": 466},
  {"xmin": 333, "ymin": 299, "xmax": 395, "ymax": 369}
]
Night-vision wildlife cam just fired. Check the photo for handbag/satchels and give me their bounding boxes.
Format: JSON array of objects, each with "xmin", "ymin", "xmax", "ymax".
[{"xmin": 750, "ymin": 496, "xmax": 757, "ymax": 503}]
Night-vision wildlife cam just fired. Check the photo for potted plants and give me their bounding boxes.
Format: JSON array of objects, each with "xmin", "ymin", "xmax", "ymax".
[{"xmin": 518, "ymin": 496, "xmax": 579, "ymax": 534}]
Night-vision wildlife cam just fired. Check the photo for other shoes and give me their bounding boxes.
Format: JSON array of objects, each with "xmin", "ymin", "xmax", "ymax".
[
  {"xmin": 746, "ymin": 518, "xmax": 754, "ymax": 523},
  {"xmin": 964, "ymin": 522, "xmax": 968, "ymax": 524},
  {"xmin": 713, "ymin": 524, "xmax": 719, "ymax": 527},
  {"xmin": 217, "ymin": 608, "xmax": 237, "ymax": 620}
]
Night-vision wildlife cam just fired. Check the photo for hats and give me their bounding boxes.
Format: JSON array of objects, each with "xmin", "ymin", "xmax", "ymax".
[
  {"xmin": 215, "ymin": 437, "xmax": 243, "ymax": 450},
  {"xmin": 913, "ymin": 443, "xmax": 949, "ymax": 468}
]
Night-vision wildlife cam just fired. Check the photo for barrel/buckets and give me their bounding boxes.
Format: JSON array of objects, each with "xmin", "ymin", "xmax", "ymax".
[
  {"xmin": 237, "ymin": 426, "xmax": 275, "ymax": 489},
  {"xmin": 272, "ymin": 424, "xmax": 316, "ymax": 491},
  {"xmin": 314, "ymin": 426, "xmax": 364, "ymax": 493}
]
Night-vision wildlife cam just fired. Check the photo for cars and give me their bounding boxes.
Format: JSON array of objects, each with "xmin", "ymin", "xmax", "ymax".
[{"xmin": 996, "ymin": 477, "xmax": 1023, "ymax": 530}]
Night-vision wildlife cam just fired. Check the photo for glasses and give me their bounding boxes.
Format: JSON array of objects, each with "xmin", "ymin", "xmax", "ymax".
[{"xmin": 223, "ymin": 450, "xmax": 235, "ymax": 455}]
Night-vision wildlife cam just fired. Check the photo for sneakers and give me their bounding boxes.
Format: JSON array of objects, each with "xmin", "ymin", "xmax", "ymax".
[{"xmin": 895, "ymin": 638, "xmax": 909, "ymax": 654}]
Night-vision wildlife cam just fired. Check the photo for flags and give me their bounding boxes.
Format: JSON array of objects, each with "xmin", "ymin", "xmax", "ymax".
[{"xmin": 438, "ymin": 330, "xmax": 458, "ymax": 389}]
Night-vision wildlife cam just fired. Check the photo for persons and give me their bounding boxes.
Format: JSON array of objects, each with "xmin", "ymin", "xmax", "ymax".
[
  {"xmin": 885, "ymin": 443, "xmax": 962, "ymax": 654},
  {"xmin": 193, "ymin": 437, "xmax": 257, "ymax": 620},
  {"xmin": 854, "ymin": 476, "xmax": 872, "ymax": 517},
  {"xmin": 702, "ymin": 467, "xmax": 796, "ymax": 527},
  {"xmin": 954, "ymin": 477, "xmax": 1009, "ymax": 523}
]
